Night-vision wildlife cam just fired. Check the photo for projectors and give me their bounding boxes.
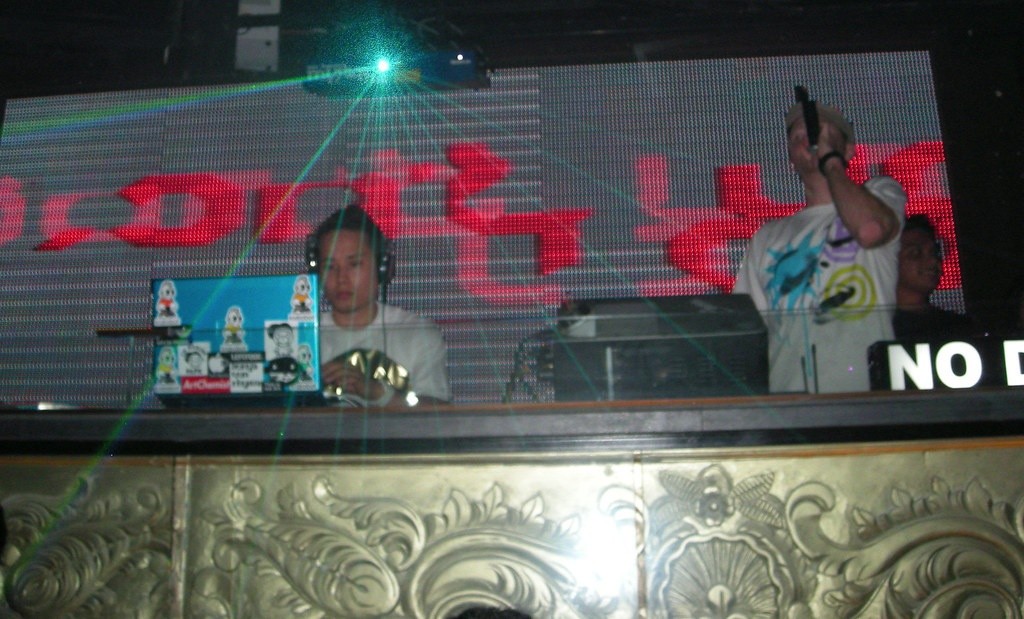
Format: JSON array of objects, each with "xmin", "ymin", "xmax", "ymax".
[{"xmin": 549, "ymin": 295, "xmax": 769, "ymax": 403}]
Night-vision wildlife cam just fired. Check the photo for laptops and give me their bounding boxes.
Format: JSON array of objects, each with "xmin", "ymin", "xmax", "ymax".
[{"xmin": 152, "ymin": 274, "xmax": 333, "ymax": 408}]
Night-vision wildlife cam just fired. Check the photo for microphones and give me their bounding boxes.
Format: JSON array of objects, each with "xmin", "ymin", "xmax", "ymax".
[{"xmin": 791, "ymin": 86, "xmax": 823, "ymax": 155}]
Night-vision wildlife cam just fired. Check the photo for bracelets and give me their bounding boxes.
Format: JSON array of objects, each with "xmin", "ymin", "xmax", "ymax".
[{"xmin": 819, "ymin": 151, "xmax": 847, "ymax": 175}]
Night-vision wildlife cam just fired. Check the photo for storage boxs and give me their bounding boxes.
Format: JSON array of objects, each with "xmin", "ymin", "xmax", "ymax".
[{"xmin": 552, "ymin": 293, "xmax": 769, "ymax": 401}]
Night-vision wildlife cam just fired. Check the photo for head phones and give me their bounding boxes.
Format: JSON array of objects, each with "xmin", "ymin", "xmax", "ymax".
[{"xmin": 304, "ymin": 231, "xmax": 397, "ymax": 287}]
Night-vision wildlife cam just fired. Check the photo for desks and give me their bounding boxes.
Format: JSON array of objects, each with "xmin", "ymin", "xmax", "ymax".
[{"xmin": 0, "ymin": 392, "xmax": 1024, "ymax": 619}]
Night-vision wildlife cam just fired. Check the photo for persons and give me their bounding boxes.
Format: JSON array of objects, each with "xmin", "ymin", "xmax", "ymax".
[
  {"xmin": 731, "ymin": 101, "xmax": 906, "ymax": 394},
  {"xmin": 307, "ymin": 204, "xmax": 451, "ymax": 410},
  {"xmin": 892, "ymin": 212, "xmax": 998, "ymax": 341}
]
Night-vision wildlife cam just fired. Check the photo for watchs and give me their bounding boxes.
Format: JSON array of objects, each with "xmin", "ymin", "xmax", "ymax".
[{"xmin": 366, "ymin": 380, "xmax": 396, "ymax": 408}]
